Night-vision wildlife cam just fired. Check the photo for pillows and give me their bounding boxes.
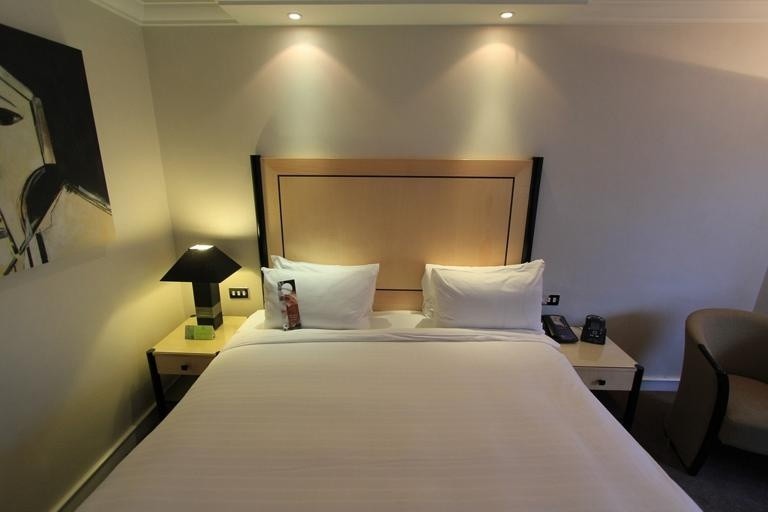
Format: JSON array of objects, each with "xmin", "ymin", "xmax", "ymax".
[
  {"xmin": 260, "ymin": 255, "xmax": 380, "ymax": 330},
  {"xmin": 412, "ymin": 257, "xmax": 546, "ymax": 335}
]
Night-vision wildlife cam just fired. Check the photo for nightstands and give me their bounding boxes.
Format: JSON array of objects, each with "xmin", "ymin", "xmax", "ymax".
[
  {"xmin": 145, "ymin": 314, "xmax": 248, "ymax": 421},
  {"xmin": 549, "ymin": 326, "xmax": 645, "ymax": 432}
]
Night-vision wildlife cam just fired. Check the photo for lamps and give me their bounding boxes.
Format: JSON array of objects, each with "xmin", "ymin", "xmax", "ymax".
[{"xmin": 159, "ymin": 243, "xmax": 242, "ymax": 331}]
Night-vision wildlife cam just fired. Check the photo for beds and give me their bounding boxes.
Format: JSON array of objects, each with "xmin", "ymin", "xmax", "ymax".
[{"xmin": 71, "ymin": 154, "xmax": 705, "ymax": 512}]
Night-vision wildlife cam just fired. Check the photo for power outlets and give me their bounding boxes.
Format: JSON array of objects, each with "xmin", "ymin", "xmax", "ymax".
[{"xmin": 229, "ymin": 288, "xmax": 248, "ymax": 298}]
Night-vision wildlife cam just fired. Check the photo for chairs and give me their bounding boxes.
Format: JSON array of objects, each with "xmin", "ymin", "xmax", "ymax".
[{"xmin": 671, "ymin": 307, "xmax": 768, "ymax": 478}]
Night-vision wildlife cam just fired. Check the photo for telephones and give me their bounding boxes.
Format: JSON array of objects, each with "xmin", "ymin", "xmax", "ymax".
[{"xmin": 542, "ymin": 315, "xmax": 578, "ymax": 343}]
